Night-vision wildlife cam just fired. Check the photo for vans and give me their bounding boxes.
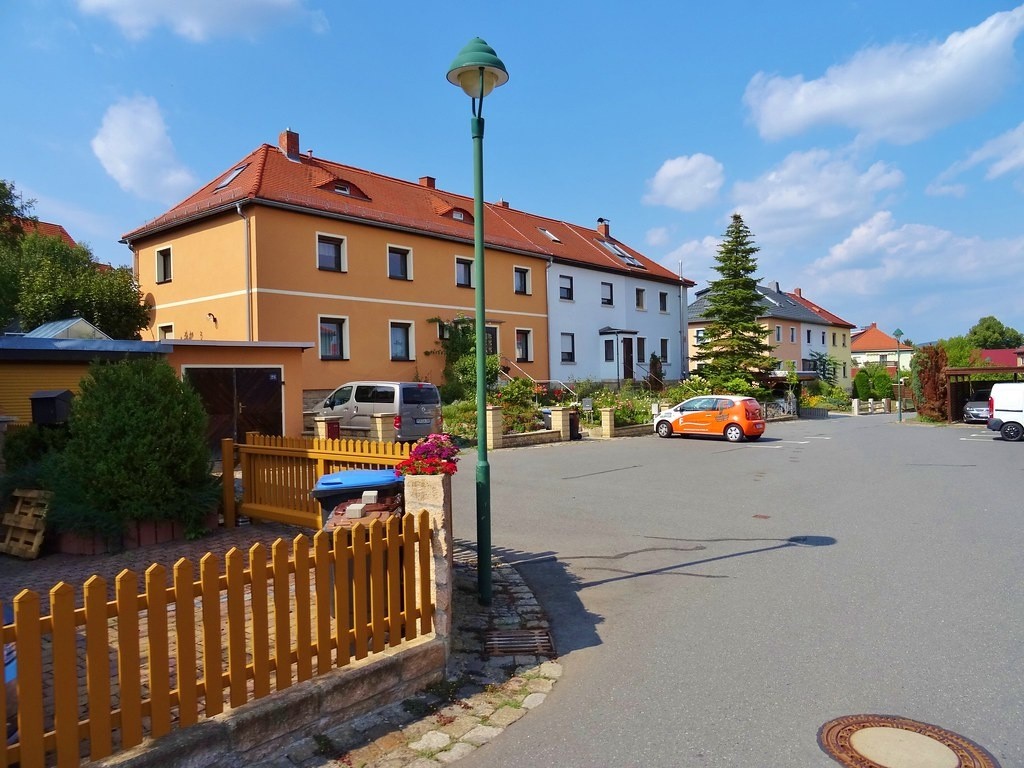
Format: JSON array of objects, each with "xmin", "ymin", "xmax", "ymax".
[
  {"xmin": 311, "ymin": 380, "xmax": 444, "ymax": 450},
  {"xmin": 986, "ymin": 382, "xmax": 1024, "ymax": 441}
]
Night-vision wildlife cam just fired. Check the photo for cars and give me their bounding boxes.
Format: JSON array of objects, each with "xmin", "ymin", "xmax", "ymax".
[
  {"xmin": 961, "ymin": 389, "xmax": 992, "ymax": 424},
  {"xmin": 654, "ymin": 394, "xmax": 766, "ymax": 443}
]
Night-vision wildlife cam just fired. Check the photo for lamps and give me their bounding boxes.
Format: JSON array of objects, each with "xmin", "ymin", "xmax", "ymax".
[{"xmin": 206, "ymin": 312, "xmax": 216, "ymax": 322}]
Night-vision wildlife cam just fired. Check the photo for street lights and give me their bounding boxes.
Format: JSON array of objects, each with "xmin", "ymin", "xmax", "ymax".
[
  {"xmin": 893, "ymin": 328, "xmax": 905, "ymax": 422},
  {"xmin": 448, "ymin": 33, "xmax": 513, "ymax": 608}
]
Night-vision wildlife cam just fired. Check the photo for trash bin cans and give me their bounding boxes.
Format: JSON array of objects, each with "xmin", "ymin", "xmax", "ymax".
[
  {"xmin": 540, "ymin": 410, "xmax": 551, "ymax": 429},
  {"xmin": 312, "ymin": 469, "xmax": 405, "ymax": 626}
]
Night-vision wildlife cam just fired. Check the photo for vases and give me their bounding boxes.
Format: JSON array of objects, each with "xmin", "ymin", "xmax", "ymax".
[{"xmin": 799, "ymin": 407, "xmax": 829, "ymax": 419}]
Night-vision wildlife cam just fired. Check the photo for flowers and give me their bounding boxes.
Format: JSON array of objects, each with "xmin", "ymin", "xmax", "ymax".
[
  {"xmin": 568, "ymin": 401, "xmax": 582, "ymax": 411},
  {"xmin": 553, "ymin": 390, "xmax": 566, "ymax": 407},
  {"xmin": 799, "ymin": 394, "xmax": 833, "ymax": 411},
  {"xmin": 393, "ymin": 433, "xmax": 461, "ymax": 479}
]
